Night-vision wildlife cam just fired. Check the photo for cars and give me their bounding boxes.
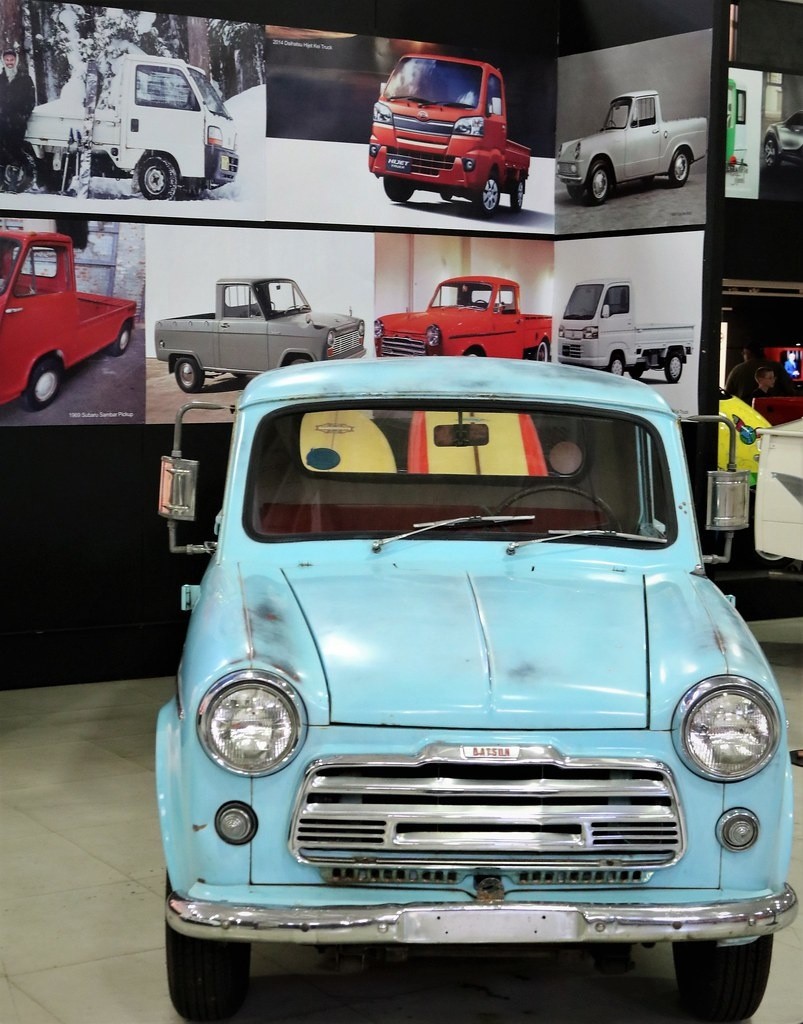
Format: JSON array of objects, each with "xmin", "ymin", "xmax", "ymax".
[
  {"xmin": 763, "ymin": 110, "xmax": 803, "ymax": 173},
  {"xmin": 150, "ymin": 356, "xmax": 802, "ymax": 1020}
]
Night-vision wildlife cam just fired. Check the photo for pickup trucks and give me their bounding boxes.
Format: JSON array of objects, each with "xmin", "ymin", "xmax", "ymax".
[
  {"xmin": 367, "ymin": 54, "xmax": 531, "ymax": 219},
  {"xmin": 2, "ymin": 53, "xmax": 239, "ymax": 201},
  {"xmin": 0, "ymin": 228, "xmax": 137, "ymax": 412},
  {"xmin": 558, "ymin": 277, "xmax": 694, "ymax": 382},
  {"xmin": 556, "ymin": 89, "xmax": 708, "ymax": 207},
  {"xmin": 374, "ymin": 275, "xmax": 553, "ymax": 362},
  {"xmin": 154, "ymin": 277, "xmax": 367, "ymax": 394}
]
